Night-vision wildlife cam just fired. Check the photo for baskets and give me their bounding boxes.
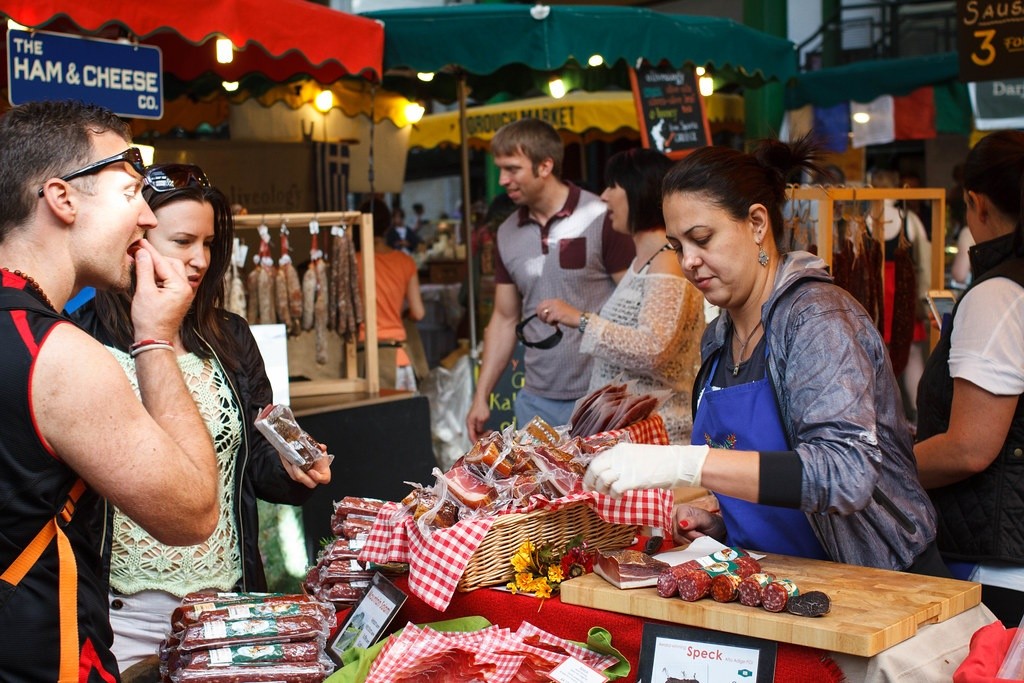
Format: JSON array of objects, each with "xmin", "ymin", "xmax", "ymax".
[{"xmin": 400, "ymin": 484, "xmax": 643, "ymax": 594}]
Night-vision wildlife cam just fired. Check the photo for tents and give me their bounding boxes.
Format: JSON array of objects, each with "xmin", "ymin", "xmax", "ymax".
[
  {"xmin": 0, "ymin": 0, "xmax": 385, "ymax": 192},
  {"xmin": 355, "ymin": 4, "xmax": 797, "ymax": 393},
  {"xmin": 784, "ymin": 51, "xmax": 972, "ymax": 152}
]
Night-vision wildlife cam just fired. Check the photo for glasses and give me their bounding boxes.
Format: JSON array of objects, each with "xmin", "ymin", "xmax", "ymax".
[
  {"xmin": 38, "ymin": 147, "xmax": 147, "ymax": 198},
  {"xmin": 515, "ymin": 313, "xmax": 564, "ymax": 350},
  {"xmin": 140, "ymin": 163, "xmax": 212, "ymax": 193}
]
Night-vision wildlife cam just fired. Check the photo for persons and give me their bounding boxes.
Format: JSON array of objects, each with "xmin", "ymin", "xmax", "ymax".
[
  {"xmin": 849, "ymin": 170, "xmax": 932, "ymax": 410},
  {"xmin": 782, "ymin": 164, "xmax": 845, "ymax": 285},
  {"xmin": 465, "ymin": 118, "xmax": 637, "ymax": 445},
  {"xmin": 339, "ymin": 199, "xmax": 425, "ymax": 391},
  {"xmin": 912, "ymin": 129, "xmax": 1024, "ymax": 629},
  {"xmin": 413, "ymin": 203, "xmax": 433, "ymax": 242},
  {"xmin": 68, "ymin": 164, "xmax": 331, "ymax": 674},
  {"xmin": 534, "ymin": 146, "xmax": 722, "ymax": 517},
  {"xmin": 585, "ymin": 145, "xmax": 951, "ymax": 580},
  {"xmin": 387, "ymin": 208, "xmax": 419, "ymax": 255},
  {"xmin": 0, "ymin": 102, "xmax": 221, "ymax": 683}
]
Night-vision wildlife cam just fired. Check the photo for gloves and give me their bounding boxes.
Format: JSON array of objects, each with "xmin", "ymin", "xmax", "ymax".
[{"xmin": 582, "ymin": 442, "xmax": 708, "ymax": 499}]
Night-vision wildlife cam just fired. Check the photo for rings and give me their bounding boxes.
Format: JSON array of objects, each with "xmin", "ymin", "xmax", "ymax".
[{"xmin": 544, "ymin": 309, "xmax": 549, "ymax": 314}]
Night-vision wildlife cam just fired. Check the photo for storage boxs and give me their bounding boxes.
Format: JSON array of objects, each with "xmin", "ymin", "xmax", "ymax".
[{"xmin": 426, "ymin": 258, "xmax": 467, "ymax": 283}]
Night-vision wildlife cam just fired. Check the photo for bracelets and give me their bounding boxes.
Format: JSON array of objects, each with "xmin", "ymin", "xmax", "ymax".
[
  {"xmin": 129, "ymin": 339, "xmax": 175, "ymax": 359},
  {"xmin": 579, "ymin": 311, "xmax": 592, "ymax": 332}
]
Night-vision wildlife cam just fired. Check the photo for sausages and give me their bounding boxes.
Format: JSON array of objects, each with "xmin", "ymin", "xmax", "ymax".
[
  {"xmin": 783, "ymin": 227, "xmax": 916, "ymax": 379},
  {"xmin": 306, "ymin": 496, "xmax": 390, "ymax": 601},
  {"xmin": 657, "ymin": 548, "xmax": 799, "ymax": 612},
  {"xmin": 159, "ymin": 591, "xmax": 336, "ymax": 683},
  {"xmin": 220, "ymin": 234, "xmax": 365, "ymax": 366}
]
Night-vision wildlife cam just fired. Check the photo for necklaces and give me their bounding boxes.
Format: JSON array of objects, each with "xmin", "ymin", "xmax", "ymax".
[{"xmin": 733, "ymin": 319, "xmax": 762, "ymax": 375}]
